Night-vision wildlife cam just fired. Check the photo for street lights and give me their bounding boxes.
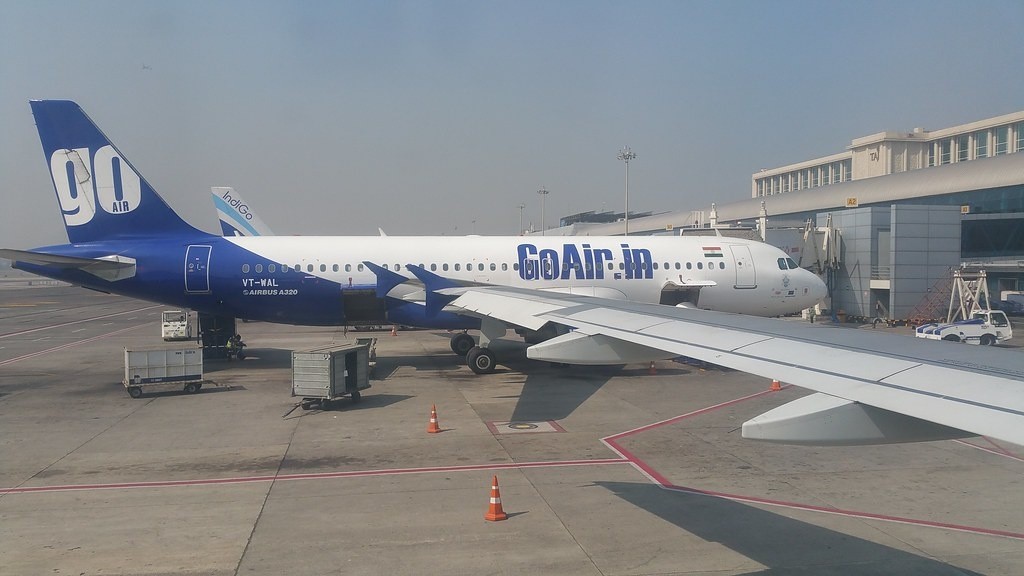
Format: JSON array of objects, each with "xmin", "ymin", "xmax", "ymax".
[
  {"xmin": 617, "ymin": 146, "xmax": 637, "ymax": 238},
  {"xmin": 517, "ymin": 204, "xmax": 526, "ymax": 237},
  {"xmin": 537, "ymin": 185, "xmax": 549, "ymax": 235}
]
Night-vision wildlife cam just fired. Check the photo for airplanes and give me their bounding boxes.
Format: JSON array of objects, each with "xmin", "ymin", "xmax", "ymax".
[
  {"xmin": 365, "ymin": 260, "xmax": 1023, "ymax": 454},
  {"xmin": 1, "ymin": 101, "xmax": 829, "ymax": 379}
]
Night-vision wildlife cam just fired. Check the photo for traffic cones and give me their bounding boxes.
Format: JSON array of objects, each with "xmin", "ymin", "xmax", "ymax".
[
  {"xmin": 426, "ymin": 403, "xmax": 442, "ymax": 434},
  {"xmin": 769, "ymin": 379, "xmax": 781, "ymax": 390},
  {"xmin": 390, "ymin": 326, "xmax": 398, "ymax": 336},
  {"xmin": 647, "ymin": 360, "xmax": 658, "ymax": 375},
  {"xmin": 484, "ymin": 474, "xmax": 508, "ymax": 522}
]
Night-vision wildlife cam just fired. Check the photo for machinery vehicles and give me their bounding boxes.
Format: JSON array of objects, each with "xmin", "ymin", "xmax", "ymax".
[{"xmin": 916, "ymin": 310, "xmax": 1013, "ymax": 346}]
